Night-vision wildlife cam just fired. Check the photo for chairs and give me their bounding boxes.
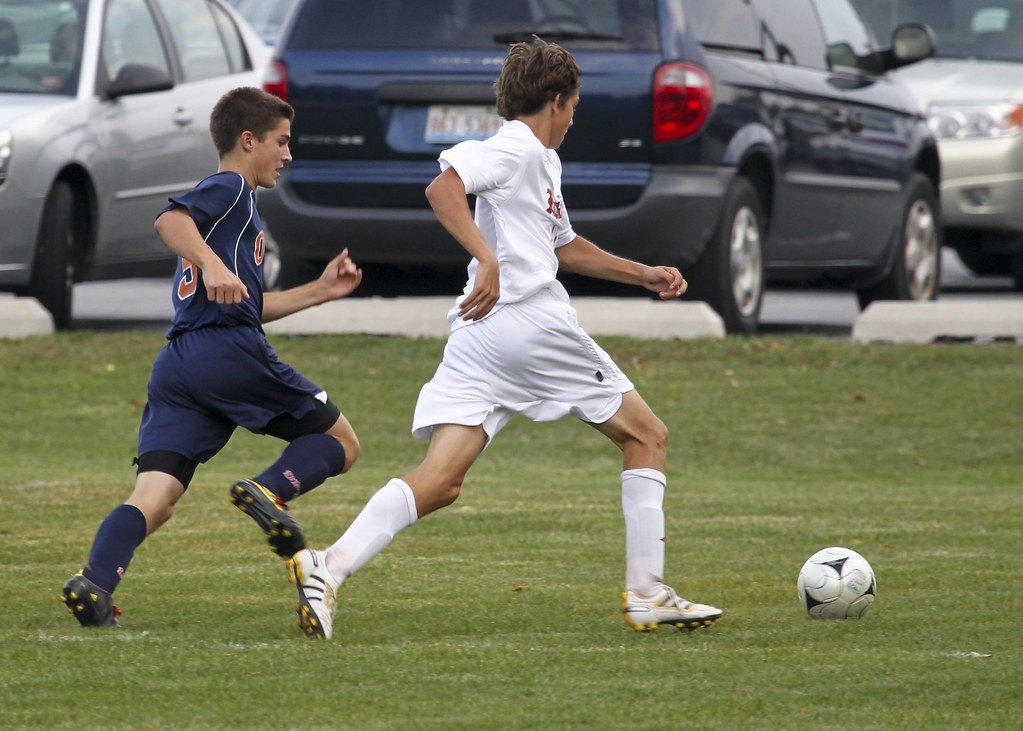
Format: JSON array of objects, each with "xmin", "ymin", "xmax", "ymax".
[{"xmin": 50, "ymin": 23, "xmax": 112, "ymax": 86}]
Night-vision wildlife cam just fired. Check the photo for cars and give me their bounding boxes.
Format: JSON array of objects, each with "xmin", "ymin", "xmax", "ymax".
[
  {"xmin": 819, "ymin": 0, "xmax": 1023, "ymax": 288},
  {"xmin": 0, "ymin": 0, "xmax": 298, "ymax": 326}
]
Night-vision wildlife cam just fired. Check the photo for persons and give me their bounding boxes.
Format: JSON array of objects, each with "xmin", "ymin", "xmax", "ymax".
[
  {"xmin": 61, "ymin": 87, "xmax": 361, "ymax": 626},
  {"xmin": 286, "ymin": 35, "xmax": 723, "ymax": 640}
]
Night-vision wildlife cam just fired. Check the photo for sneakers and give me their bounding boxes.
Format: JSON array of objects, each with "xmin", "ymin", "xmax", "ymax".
[
  {"xmin": 228, "ymin": 478, "xmax": 306, "ymax": 559},
  {"xmin": 287, "ymin": 547, "xmax": 340, "ymax": 640},
  {"xmin": 619, "ymin": 582, "xmax": 723, "ymax": 632},
  {"xmin": 59, "ymin": 568, "xmax": 122, "ymax": 628}
]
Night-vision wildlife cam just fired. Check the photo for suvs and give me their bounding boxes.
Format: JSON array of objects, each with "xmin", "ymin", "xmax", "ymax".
[{"xmin": 257, "ymin": 0, "xmax": 948, "ymax": 331}]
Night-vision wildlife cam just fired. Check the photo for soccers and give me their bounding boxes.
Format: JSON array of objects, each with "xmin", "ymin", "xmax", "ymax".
[{"xmin": 796, "ymin": 545, "xmax": 877, "ymax": 621}]
